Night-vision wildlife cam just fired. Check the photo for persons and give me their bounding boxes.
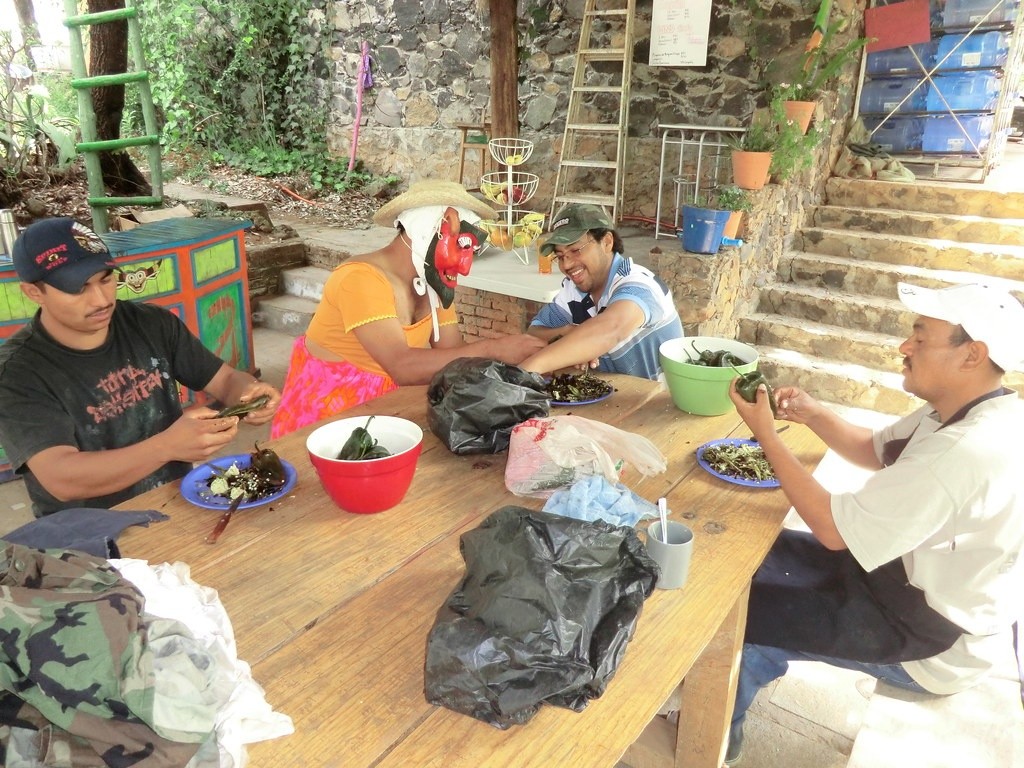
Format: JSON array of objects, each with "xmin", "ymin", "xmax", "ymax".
[
  {"xmin": 0, "ymin": 217, "xmax": 281, "ymax": 521},
  {"xmin": 517, "ymin": 204, "xmax": 684, "ymax": 389},
  {"xmin": 656, "ymin": 281, "xmax": 1023, "ymax": 768},
  {"xmin": 270, "ymin": 182, "xmax": 548, "ymax": 442}
]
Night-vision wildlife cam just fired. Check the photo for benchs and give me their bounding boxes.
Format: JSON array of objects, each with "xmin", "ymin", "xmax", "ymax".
[{"xmin": 845, "ymin": 633, "xmax": 1024, "ymax": 768}]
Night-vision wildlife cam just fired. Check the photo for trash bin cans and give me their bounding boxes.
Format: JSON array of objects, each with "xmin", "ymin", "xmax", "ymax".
[{"xmin": 64, "ymin": 0, "xmax": 163, "ymax": 235}]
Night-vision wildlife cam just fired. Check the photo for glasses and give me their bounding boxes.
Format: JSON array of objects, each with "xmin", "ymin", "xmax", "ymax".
[{"xmin": 551, "ymin": 239, "xmax": 593, "ymax": 265}]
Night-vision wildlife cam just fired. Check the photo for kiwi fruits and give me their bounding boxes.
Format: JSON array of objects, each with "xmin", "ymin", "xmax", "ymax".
[{"xmin": 497, "ymin": 220, "xmax": 506, "ymax": 230}]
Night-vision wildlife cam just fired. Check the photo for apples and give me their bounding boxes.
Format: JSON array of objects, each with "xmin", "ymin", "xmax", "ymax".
[
  {"xmin": 502, "ymin": 186, "xmax": 527, "ymax": 202},
  {"xmin": 514, "ymin": 231, "xmax": 531, "ymax": 247},
  {"xmin": 510, "ymin": 226, "xmax": 520, "ymax": 236}
]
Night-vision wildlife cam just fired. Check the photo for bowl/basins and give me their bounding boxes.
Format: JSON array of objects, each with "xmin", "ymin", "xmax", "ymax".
[
  {"xmin": 659, "ymin": 336, "xmax": 761, "ymax": 417},
  {"xmin": 306, "ymin": 415, "xmax": 424, "ymax": 515}
]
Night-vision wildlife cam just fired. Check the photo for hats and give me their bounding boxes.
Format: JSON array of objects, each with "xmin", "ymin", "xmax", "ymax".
[
  {"xmin": 13, "ymin": 217, "xmax": 124, "ymax": 294},
  {"xmin": 538, "ymin": 204, "xmax": 615, "ymax": 257},
  {"xmin": 897, "ymin": 282, "xmax": 1023, "ymax": 373},
  {"xmin": 374, "ymin": 180, "xmax": 500, "ymax": 227}
]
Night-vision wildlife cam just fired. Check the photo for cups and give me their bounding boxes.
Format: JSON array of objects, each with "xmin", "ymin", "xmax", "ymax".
[
  {"xmin": 538, "ymin": 237, "xmax": 553, "ymax": 275},
  {"xmin": 635, "ymin": 520, "xmax": 693, "ymax": 589}
]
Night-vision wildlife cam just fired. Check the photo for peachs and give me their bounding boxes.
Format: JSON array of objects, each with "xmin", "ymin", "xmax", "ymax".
[{"xmin": 497, "ymin": 193, "xmax": 507, "ymax": 204}]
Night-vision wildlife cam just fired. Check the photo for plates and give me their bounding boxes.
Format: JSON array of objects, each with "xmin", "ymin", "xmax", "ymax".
[
  {"xmin": 696, "ymin": 438, "xmax": 779, "ymax": 488},
  {"xmin": 539, "ymin": 377, "xmax": 614, "ymax": 405},
  {"xmin": 180, "ymin": 454, "xmax": 297, "ymax": 510}
]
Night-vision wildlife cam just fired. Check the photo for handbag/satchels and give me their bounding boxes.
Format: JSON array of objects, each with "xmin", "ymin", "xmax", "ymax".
[{"xmin": 503, "ymin": 415, "xmax": 667, "ymax": 499}]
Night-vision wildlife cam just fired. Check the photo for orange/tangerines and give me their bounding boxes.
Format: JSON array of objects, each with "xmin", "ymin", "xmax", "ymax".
[{"xmin": 491, "ymin": 230, "xmax": 507, "ymax": 246}]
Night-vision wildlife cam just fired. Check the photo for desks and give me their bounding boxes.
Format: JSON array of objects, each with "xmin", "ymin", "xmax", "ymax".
[{"xmin": 98, "ymin": 371, "xmax": 828, "ymax": 768}]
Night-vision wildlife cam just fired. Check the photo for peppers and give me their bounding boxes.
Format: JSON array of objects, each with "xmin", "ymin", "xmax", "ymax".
[
  {"xmin": 335, "ymin": 415, "xmax": 392, "ymax": 460},
  {"xmin": 215, "ymin": 394, "xmax": 270, "ymax": 418},
  {"xmin": 549, "ymin": 372, "xmax": 611, "ymax": 403},
  {"xmin": 683, "ymin": 340, "xmax": 748, "ymax": 366},
  {"xmin": 250, "ymin": 440, "xmax": 287, "ymax": 487},
  {"xmin": 728, "ymin": 361, "xmax": 776, "ymax": 413}
]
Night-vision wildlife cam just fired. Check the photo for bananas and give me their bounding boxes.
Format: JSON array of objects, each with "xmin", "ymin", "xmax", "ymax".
[
  {"xmin": 480, "ymin": 181, "xmax": 513, "ymax": 196},
  {"xmin": 480, "ymin": 220, "xmax": 496, "ymax": 241},
  {"xmin": 518, "ymin": 214, "xmax": 544, "ymax": 235}
]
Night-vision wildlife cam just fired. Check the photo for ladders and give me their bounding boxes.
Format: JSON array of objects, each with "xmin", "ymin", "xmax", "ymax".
[{"xmin": 548, "ymin": 0, "xmax": 636, "ymax": 233}]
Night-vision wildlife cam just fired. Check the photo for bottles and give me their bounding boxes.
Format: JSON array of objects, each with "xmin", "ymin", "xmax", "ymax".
[{"xmin": 0, "ymin": 209, "xmax": 20, "ymax": 262}]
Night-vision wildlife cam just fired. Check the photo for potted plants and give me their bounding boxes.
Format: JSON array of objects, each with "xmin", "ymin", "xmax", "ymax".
[
  {"xmin": 681, "ymin": 197, "xmax": 731, "ymax": 254},
  {"xmin": 727, "ymin": 117, "xmax": 782, "ymax": 190},
  {"xmin": 773, "ymin": 16, "xmax": 879, "ymax": 136},
  {"xmin": 707, "ymin": 188, "xmax": 753, "ymax": 239}
]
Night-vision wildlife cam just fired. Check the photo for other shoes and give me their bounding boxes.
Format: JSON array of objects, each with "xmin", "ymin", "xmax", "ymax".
[{"xmin": 725, "ymin": 735, "xmax": 741, "ymax": 763}]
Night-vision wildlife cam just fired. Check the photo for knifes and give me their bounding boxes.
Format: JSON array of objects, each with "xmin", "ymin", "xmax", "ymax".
[
  {"xmin": 751, "ymin": 425, "xmax": 790, "ymax": 442},
  {"xmin": 205, "ymin": 492, "xmax": 246, "ymax": 544}
]
